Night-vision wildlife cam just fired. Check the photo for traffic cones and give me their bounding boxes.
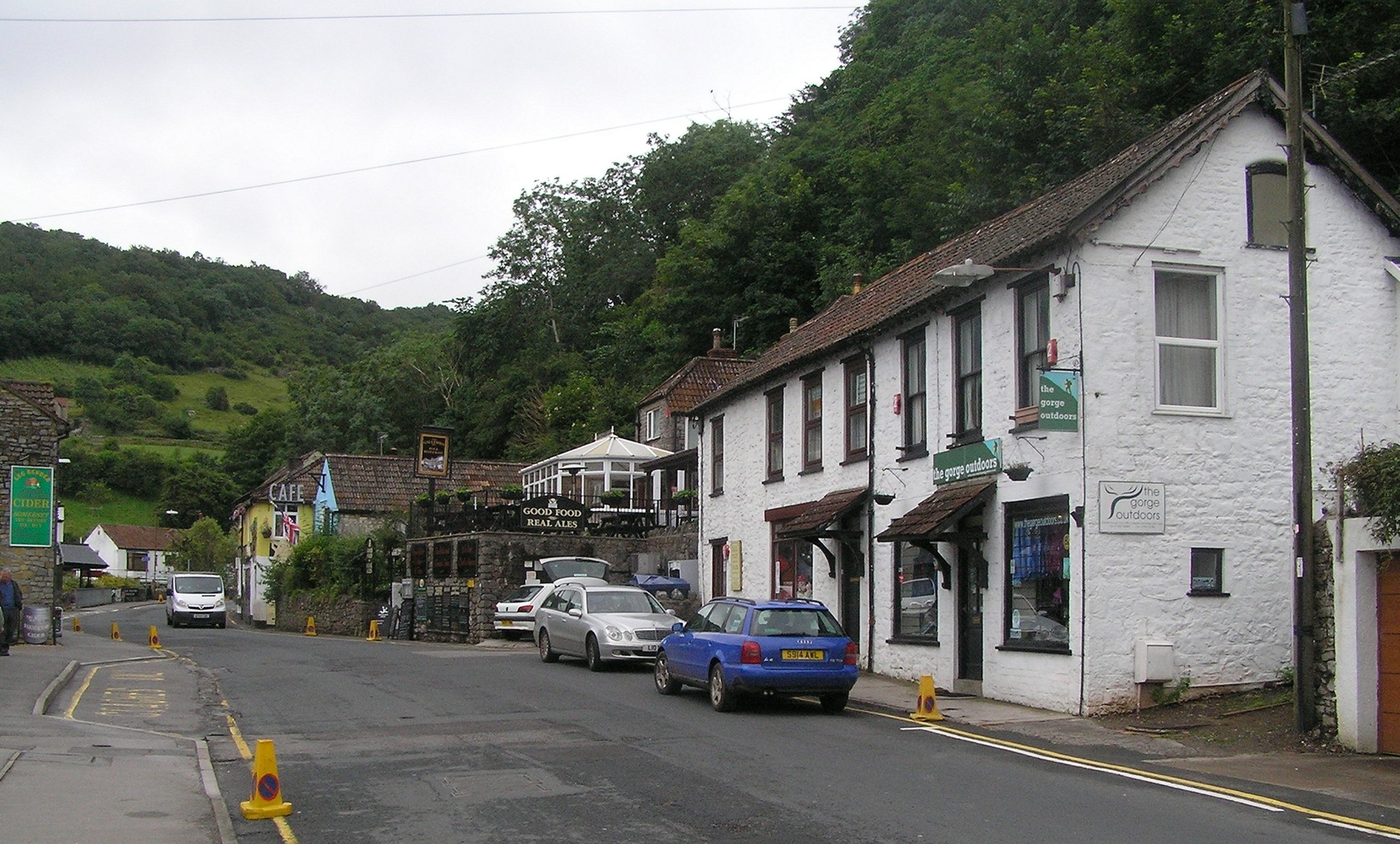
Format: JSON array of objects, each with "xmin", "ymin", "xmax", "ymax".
[
  {"xmin": 238, "ymin": 739, "xmax": 292, "ymax": 820},
  {"xmin": 111, "ymin": 622, "xmax": 123, "ymax": 641},
  {"xmin": 303, "ymin": 616, "xmax": 319, "ymax": 637},
  {"xmin": 73, "ymin": 616, "xmax": 84, "ymax": 634},
  {"xmin": 147, "ymin": 627, "xmax": 161, "ymax": 649},
  {"xmin": 364, "ymin": 620, "xmax": 383, "ymax": 641},
  {"xmin": 158, "ymin": 593, "xmax": 164, "ymax": 603}
]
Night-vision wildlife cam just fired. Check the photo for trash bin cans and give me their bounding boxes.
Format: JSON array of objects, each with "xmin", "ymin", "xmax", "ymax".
[{"xmin": 22, "ymin": 605, "xmax": 51, "ymax": 644}]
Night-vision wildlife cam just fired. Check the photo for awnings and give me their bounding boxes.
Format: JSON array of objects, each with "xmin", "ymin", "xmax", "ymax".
[
  {"xmin": 872, "ymin": 479, "xmax": 993, "ymax": 590},
  {"xmin": 777, "ymin": 486, "xmax": 869, "ymax": 577}
]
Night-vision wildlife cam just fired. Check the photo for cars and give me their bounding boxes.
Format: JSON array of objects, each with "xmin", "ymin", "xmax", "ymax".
[
  {"xmin": 899, "ymin": 575, "xmax": 938, "ymax": 620},
  {"xmin": 493, "ymin": 582, "xmax": 556, "ymax": 647},
  {"xmin": 1010, "ymin": 593, "xmax": 1068, "ymax": 644}
]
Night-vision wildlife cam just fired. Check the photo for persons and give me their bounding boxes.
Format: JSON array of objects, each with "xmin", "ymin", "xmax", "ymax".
[{"xmin": 0, "ymin": 568, "xmax": 23, "ymax": 644}]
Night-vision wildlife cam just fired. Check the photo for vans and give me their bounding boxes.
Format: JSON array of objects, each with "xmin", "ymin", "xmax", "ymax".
[{"xmin": 164, "ymin": 572, "xmax": 229, "ymax": 628}]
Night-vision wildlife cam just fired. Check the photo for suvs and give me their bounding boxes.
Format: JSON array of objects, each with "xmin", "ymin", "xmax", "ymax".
[
  {"xmin": 653, "ymin": 597, "xmax": 861, "ymax": 716},
  {"xmin": 531, "ymin": 554, "xmax": 685, "ymax": 673}
]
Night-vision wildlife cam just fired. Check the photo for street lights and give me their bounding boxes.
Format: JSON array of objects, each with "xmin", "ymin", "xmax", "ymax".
[
  {"xmin": 153, "ymin": 504, "xmax": 179, "ymax": 599},
  {"xmin": 930, "ymin": 257, "xmax": 1063, "ymax": 289}
]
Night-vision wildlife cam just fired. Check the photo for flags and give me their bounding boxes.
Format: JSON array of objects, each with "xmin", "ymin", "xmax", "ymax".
[{"xmin": 281, "ymin": 511, "xmax": 300, "ymax": 545}]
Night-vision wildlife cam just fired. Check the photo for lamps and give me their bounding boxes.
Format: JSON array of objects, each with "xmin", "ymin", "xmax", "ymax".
[{"xmin": 930, "ymin": 257, "xmax": 1068, "ymax": 303}]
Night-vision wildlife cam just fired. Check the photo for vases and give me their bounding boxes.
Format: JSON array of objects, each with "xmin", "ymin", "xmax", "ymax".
[
  {"xmin": 1003, "ymin": 467, "xmax": 1034, "ymax": 481},
  {"xmin": 872, "ymin": 494, "xmax": 896, "ymax": 505}
]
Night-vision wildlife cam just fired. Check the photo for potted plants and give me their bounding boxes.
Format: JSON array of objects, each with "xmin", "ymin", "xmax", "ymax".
[
  {"xmin": 499, "ymin": 482, "xmax": 523, "ymax": 499},
  {"xmin": 601, "ymin": 489, "xmax": 625, "ymax": 505},
  {"xmin": 456, "ymin": 484, "xmax": 472, "ymax": 502},
  {"xmin": 435, "ymin": 489, "xmax": 451, "ymax": 505},
  {"xmin": 413, "ymin": 491, "xmax": 433, "ymax": 508},
  {"xmin": 672, "ymin": 490, "xmax": 692, "ymax": 505}
]
